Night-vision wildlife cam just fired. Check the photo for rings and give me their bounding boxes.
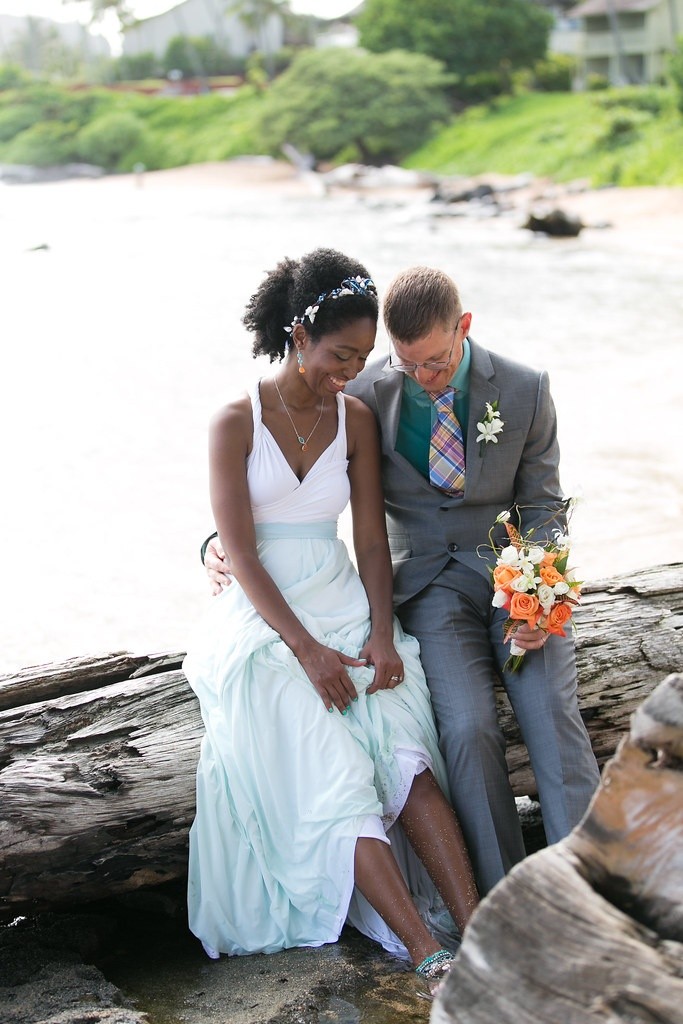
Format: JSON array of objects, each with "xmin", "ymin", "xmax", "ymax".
[
  {"xmin": 391, "ymin": 676, "xmax": 398, "ymax": 681},
  {"xmin": 398, "ymin": 677, "xmax": 402, "ymax": 681},
  {"xmin": 542, "ymin": 638, "xmax": 545, "ymax": 643}
]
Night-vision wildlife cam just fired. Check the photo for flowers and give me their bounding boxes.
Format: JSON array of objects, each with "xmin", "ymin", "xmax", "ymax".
[
  {"xmin": 476, "ymin": 498, "xmax": 584, "ymax": 675},
  {"xmin": 478, "ymin": 400, "xmax": 507, "ymax": 445}
]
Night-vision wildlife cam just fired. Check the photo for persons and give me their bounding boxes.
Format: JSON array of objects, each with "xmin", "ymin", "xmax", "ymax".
[
  {"xmin": 200, "ymin": 264, "xmax": 600, "ymax": 903},
  {"xmin": 183, "ymin": 249, "xmax": 478, "ymax": 981}
]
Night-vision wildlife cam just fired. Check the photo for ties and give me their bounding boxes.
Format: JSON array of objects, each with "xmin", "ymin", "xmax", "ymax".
[{"xmin": 425, "ymin": 386, "xmax": 465, "ymax": 497}]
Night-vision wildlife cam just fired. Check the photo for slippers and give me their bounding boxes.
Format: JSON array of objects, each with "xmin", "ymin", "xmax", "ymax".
[{"xmin": 405, "ymin": 950, "xmax": 455, "ymax": 982}]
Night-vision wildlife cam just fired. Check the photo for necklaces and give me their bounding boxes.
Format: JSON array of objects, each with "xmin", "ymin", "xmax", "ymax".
[{"xmin": 273, "ymin": 377, "xmax": 324, "ymax": 451}]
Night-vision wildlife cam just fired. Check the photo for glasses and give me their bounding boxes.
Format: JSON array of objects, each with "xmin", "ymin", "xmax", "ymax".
[{"xmin": 389, "ymin": 317, "xmax": 461, "ymax": 372}]
